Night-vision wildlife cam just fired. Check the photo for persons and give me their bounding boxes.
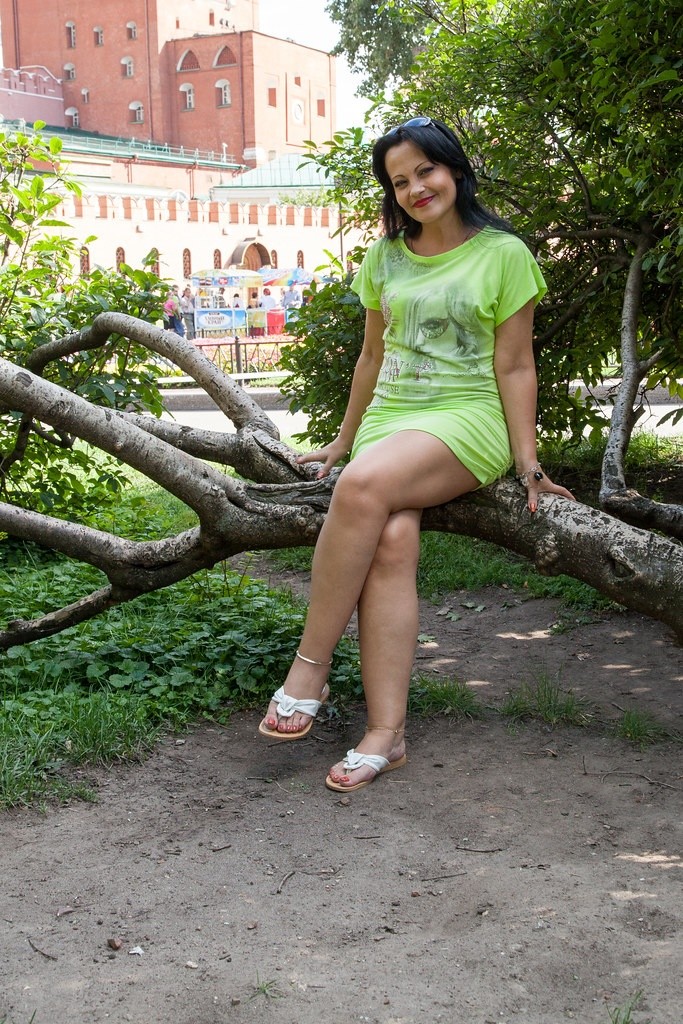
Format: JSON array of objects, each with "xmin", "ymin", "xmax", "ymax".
[
  {"xmin": 165, "ymin": 284, "xmax": 302, "ymax": 339},
  {"xmin": 257, "ymin": 119, "xmax": 573, "ymax": 792}
]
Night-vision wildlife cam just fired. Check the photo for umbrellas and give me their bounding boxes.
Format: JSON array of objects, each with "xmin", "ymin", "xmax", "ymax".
[{"xmin": 257, "ymin": 268, "xmax": 338, "ymax": 286}]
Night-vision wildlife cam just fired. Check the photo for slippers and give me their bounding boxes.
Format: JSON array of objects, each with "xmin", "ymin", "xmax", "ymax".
[
  {"xmin": 326, "ymin": 748, "xmax": 407, "ymax": 792},
  {"xmin": 258, "ymin": 682, "xmax": 330, "ymax": 739}
]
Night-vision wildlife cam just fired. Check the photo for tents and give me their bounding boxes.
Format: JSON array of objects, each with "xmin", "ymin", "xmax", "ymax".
[{"xmin": 191, "ymin": 269, "xmax": 262, "ymax": 287}]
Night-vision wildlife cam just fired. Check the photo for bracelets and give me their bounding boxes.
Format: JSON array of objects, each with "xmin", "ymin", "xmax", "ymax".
[{"xmin": 516, "ymin": 463, "xmax": 543, "ymax": 487}]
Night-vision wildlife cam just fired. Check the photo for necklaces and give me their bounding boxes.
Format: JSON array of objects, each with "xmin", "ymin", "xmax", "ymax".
[{"xmin": 411, "ymin": 225, "xmax": 475, "ymax": 255}]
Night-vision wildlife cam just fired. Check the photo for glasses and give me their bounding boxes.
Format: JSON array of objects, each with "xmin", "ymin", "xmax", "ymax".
[{"xmin": 384, "ymin": 116, "xmax": 432, "ymax": 136}]
